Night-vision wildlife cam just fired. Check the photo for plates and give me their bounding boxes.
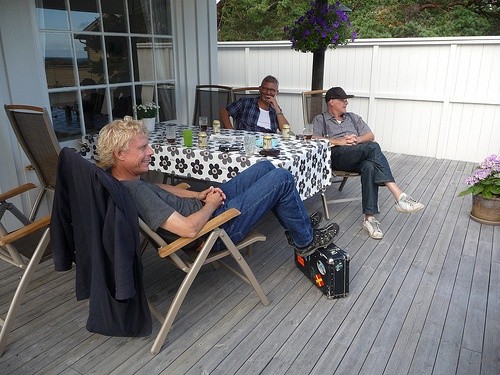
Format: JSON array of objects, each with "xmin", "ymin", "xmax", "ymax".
[{"xmin": 255, "ymin": 138, "xmax": 279, "ymax": 148}]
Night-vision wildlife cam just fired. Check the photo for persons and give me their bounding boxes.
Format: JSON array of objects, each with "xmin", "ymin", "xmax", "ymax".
[
  {"xmin": 311, "ymin": 87, "xmax": 424, "ymax": 239},
  {"xmin": 80, "ymin": 72, "xmax": 132, "ymax": 130},
  {"xmin": 96, "ymin": 115, "xmax": 339, "ymax": 256},
  {"xmin": 220, "ymin": 75, "xmax": 290, "ymax": 134}
]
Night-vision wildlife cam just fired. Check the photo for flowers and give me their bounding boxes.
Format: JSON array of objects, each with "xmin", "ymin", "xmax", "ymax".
[
  {"xmin": 284, "ymin": 0, "xmax": 359, "ymax": 53},
  {"xmin": 455, "ymin": 153, "xmax": 500, "ymax": 200},
  {"xmin": 132, "ymin": 101, "xmax": 160, "ymax": 120}
]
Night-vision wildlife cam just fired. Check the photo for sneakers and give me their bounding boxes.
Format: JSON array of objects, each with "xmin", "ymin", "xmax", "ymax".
[
  {"xmin": 285, "ymin": 211, "xmax": 322, "ymax": 248},
  {"xmin": 294, "ymin": 223, "xmax": 339, "ymax": 256},
  {"xmin": 362, "ymin": 216, "xmax": 383, "ymax": 239},
  {"xmin": 395, "ymin": 195, "xmax": 425, "ymax": 213}
]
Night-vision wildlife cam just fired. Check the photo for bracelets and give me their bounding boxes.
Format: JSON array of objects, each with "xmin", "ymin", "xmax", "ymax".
[{"xmin": 276, "ymin": 112, "xmax": 283, "ymax": 114}]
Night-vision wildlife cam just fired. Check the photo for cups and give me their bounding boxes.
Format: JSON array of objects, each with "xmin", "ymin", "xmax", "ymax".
[
  {"xmin": 199, "ymin": 117, "xmax": 208, "ymax": 131},
  {"xmin": 244, "ymin": 136, "xmax": 256, "ymax": 157},
  {"xmin": 303, "ymin": 123, "xmax": 313, "ymax": 140},
  {"xmin": 166, "ymin": 126, "xmax": 176, "ymax": 144}
]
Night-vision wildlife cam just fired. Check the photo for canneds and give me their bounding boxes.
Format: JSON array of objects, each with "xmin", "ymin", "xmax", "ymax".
[
  {"xmin": 263, "ymin": 136, "xmax": 273, "ymax": 150},
  {"xmin": 213, "ymin": 120, "xmax": 220, "ymax": 134},
  {"xmin": 282, "ymin": 124, "xmax": 290, "ymax": 139},
  {"xmin": 198, "ymin": 132, "xmax": 207, "ymax": 149}
]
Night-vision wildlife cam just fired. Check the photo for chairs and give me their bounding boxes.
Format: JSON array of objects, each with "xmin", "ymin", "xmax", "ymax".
[
  {"xmin": 302, "ymin": 89, "xmax": 381, "ymax": 220},
  {"xmin": 192, "ymin": 84, "xmax": 233, "ymax": 128},
  {"xmin": 0, "ymin": 182, "xmax": 51, "ymax": 352},
  {"xmin": 153, "ymin": 84, "xmax": 176, "ymax": 122},
  {"xmin": 3, "ymin": 104, "xmax": 62, "ymax": 220},
  {"xmin": 50, "ymin": 147, "xmax": 270, "ymax": 355},
  {"xmin": 231, "ymin": 87, "xmax": 260, "ymax": 127}
]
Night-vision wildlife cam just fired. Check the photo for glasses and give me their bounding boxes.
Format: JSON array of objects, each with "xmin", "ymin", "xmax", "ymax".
[{"xmin": 261, "ymin": 86, "xmax": 277, "ymax": 93}]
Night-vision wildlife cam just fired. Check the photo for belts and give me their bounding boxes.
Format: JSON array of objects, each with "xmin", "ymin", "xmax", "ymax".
[{"xmin": 188, "ymin": 240, "xmax": 203, "ymax": 252}]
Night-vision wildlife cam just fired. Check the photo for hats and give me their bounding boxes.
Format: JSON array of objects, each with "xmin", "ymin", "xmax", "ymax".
[{"xmin": 325, "ymin": 87, "xmax": 354, "ymax": 102}]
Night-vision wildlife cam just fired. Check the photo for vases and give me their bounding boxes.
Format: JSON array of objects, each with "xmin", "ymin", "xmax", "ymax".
[
  {"xmin": 141, "ymin": 117, "xmax": 155, "ymax": 132},
  {"xmin": 468, "ymin": 193, "xmax": 500, "ymax": 226}
]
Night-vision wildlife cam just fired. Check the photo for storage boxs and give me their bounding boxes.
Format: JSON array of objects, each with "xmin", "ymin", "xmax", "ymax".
[{"xmin": 293, "ymin": 241, "xmax": 350, "ymax": 300}]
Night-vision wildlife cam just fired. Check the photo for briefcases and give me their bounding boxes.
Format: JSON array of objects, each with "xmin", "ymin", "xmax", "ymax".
[{"xmin": 295, "ymin": 242, "xmax": 349, "ymax": 299}]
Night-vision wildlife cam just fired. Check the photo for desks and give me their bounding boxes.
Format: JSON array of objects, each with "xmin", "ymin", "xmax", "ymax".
[
  {"xmin": 52, "ymin": 107, "xmax": 81, "ymax": 138},
  {"xmin": 79, "ymin": 121, "xmax": 333, "ymax": 258}
]
